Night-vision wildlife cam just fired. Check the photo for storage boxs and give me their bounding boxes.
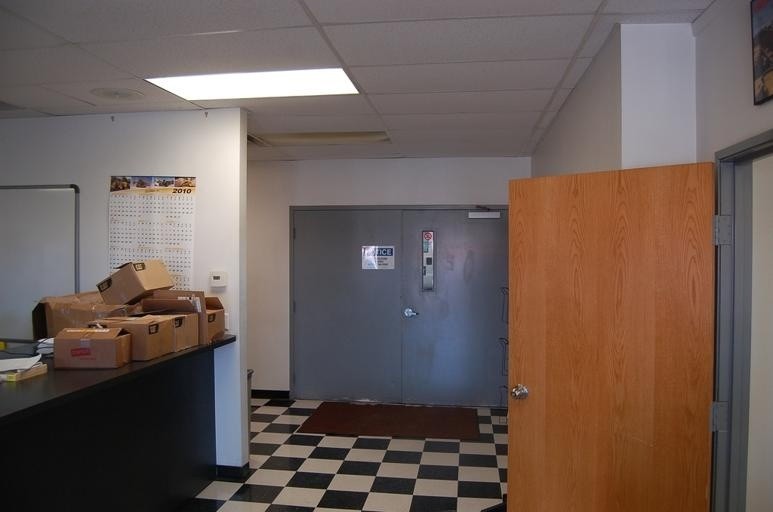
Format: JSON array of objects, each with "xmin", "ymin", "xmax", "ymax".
[{"xmin": 35, "ymin": 255, "xmax": 228, "ymax": 372}]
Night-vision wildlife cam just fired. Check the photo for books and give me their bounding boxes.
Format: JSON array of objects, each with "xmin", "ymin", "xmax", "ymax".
[{"xmin": 0, "ymin": 362, "xmax": 47, "ymax": 381}]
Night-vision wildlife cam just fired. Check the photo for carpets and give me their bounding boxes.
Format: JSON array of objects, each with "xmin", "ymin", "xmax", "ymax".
[{"xmin": 296, "ymin": 401, "xmax": 483, "ymax": 444}]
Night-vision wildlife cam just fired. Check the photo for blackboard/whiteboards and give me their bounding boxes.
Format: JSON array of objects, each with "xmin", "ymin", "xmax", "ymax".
[{"xmin": 0, "ymin": 184, "xmax": 80, "ymax": 343}]
